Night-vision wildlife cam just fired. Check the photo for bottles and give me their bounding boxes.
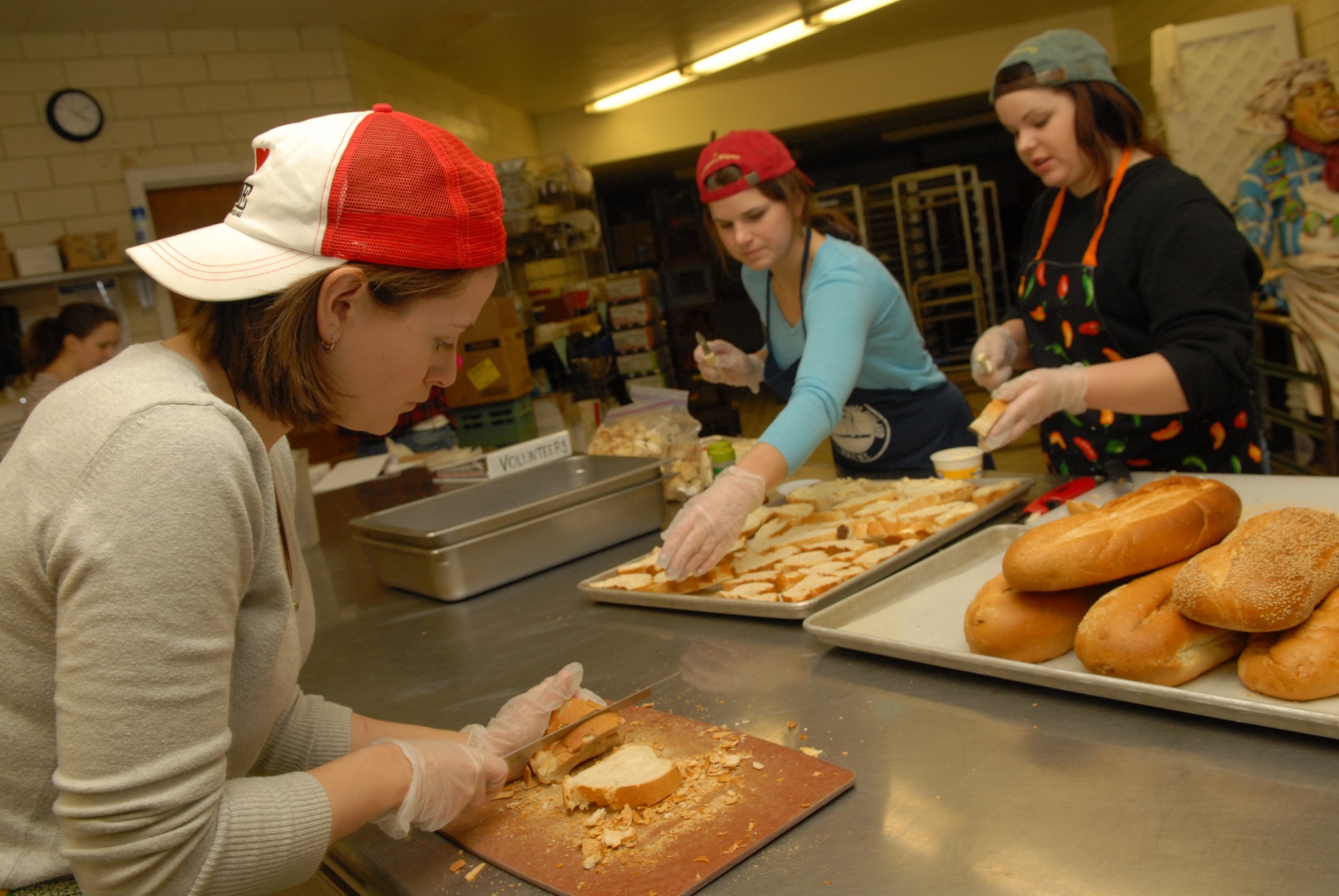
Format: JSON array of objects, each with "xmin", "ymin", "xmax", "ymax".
[
  {"xmin": 706, "ymin": 437, "xmax": 733, "ymax": 457},
  {"xmin": 708, "ymin": 446, "xmax": 736, "ymax": 479}
]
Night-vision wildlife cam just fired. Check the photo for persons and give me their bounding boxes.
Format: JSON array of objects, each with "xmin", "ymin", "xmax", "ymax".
[
  {"xmin": 1, "ymin": 101, "xmax": 606, "ymax": 896},
  {"xmin": 654, "ymin": 128, "xmax": 996, "ymax": 589},
  {"xmin": 960, "ymin": 28, "xmax": 1265, "ymax": 475},
  {"xmin": 0, "ymin": 304, "xmax": 119, "ymax": 472}
]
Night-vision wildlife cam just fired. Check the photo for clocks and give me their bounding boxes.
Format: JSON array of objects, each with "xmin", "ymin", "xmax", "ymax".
[{"xmin": 46, "ymin": 88, "xmax": 103, "ymax": 142}]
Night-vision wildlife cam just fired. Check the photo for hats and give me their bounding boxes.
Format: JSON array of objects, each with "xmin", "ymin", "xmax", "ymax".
[
  {"xmin": 125, "ymin": 104, "xmax": 507, "ymax": 302},
  {"xmin": 989, "ymin": 28, "xmax": 1144, "ymax": 138},
  {"xmin": 695, "ymin": 130, "xmax": 813, "ymax": 215}
]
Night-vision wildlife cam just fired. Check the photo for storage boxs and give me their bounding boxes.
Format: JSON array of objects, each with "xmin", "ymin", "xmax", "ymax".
[
  {"xmin": 443, "ymin": 256, "xmax": 680, "ymax": 445},
  {"xmin": 0, "ymin": 232, "xmax": 14, "ymax": 281},
  {"xmin": 11, "ymin": 245, "xmax": 63, "ymax": 277},
  {"xmin": 58, "ymin": 230, "xmax": 121, "ymax": 271}
]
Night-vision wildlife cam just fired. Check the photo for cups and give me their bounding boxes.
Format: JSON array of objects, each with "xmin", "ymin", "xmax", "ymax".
[{"xmin": 929, "ymin": 446, "xmax": 986, "ymax": 480}]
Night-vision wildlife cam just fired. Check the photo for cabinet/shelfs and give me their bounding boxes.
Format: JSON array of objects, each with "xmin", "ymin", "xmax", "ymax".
[
  {"xmin": 812, "ymin": 162, "xmax": 1007, "ymax": 367},
  {"xmin": 491, "ymin": 150, "xmax": 609, "ymax": 290}
]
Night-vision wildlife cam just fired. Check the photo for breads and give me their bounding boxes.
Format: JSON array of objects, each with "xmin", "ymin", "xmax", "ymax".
[
  {"xmin": 962, "ymin": 473, "xmax": 1339, "ymax": 702},
  {"xmin": 586, "ymin": 470, "xmax": 1022, "ymax": 605},
  {"xmin": 528, "ymin": 700, "xmax": 681, "ymax": 811},
  {"xmin": 587, "ymin": 417, "xmax": 713, "ymax": 501}
]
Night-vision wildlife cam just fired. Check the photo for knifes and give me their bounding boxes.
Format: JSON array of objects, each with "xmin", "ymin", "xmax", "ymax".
[
  {"xmin": 497, "ymin": 670, "xmax": 684, "ymax": 784},
  {"xmin": 695, "ymin": 332, "xmax": 725, "ymax": 382}
]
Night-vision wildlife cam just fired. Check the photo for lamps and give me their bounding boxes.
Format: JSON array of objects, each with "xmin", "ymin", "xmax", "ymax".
[{"xmin": 583, "ymin": 0, "xmax": 898, "ymax": 112}]
